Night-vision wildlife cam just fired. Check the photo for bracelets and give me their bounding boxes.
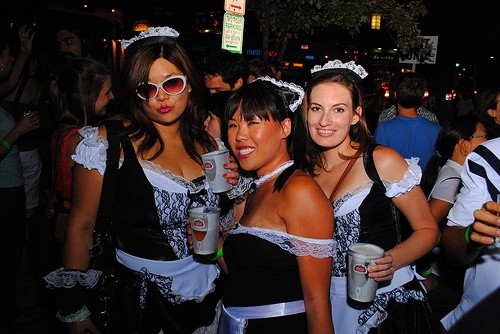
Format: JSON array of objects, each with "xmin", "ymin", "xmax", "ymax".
[
  {"xmin": 465, "ymin": 224, "xmax": 484, "ymax": 249},
  {"xmin": 211, "ymin": 248, "xmax": 223, "ymax": 261},
  {"xmin": 0, "ymin": 138, "xmax": 12, "ymax": 150},
  {"xmin": 420, "ymin": 268, "xmax": 432, "ymax": 277}
]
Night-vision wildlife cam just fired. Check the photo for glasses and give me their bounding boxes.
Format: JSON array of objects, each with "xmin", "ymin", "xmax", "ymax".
[
  {"xmin": 464, "ymin": 133, "xmax": 489, "ymax": 142},
  {"xmin": 136, "ymin": 75, "xmax": 187, "ymax": 101}
]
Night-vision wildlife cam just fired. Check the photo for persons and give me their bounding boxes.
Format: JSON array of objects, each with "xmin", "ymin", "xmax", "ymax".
[
  {"xmin": 188, "ymin": 75, "xmax": 334, "ymax": 334},
  {"xmin": 0, "ymin": 22, "xmax": 500, "ymax": 334}
]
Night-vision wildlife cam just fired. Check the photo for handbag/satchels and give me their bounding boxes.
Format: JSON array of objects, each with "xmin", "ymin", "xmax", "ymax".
[
  {"xmin": 80, "ymin": 119, "xmax": 123, "ymax": 334},
  {"xmin": 55, "ymin": 212, "xmax": 70, "ymax": 241}
]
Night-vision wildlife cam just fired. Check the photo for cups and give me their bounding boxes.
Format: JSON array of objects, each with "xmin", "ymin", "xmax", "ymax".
[
  {"xmin": 188, "ymin": 207, "xmax": 221, "ymax": 262},
  {"xmin": 201, "ymin": 149, "xmax": 232, "ymax": 193},
  {"xmin": 347, "ymin": 243, "xmax": 385, "ymax": 302}
]
